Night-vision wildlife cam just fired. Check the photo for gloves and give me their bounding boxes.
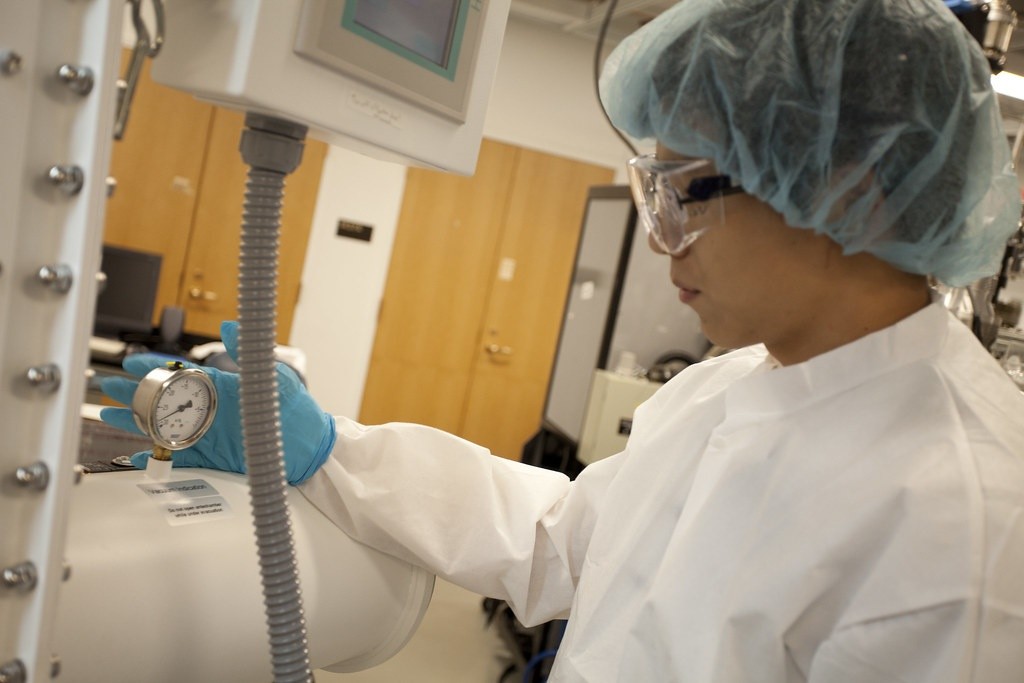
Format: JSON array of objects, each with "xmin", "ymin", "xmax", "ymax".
[{"xmin": 99, "ymin": 321, "xmax": 337, "ymax": 487}]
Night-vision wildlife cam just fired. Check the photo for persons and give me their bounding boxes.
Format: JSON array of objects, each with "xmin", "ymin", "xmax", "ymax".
[{"xmin": 96, "ymin": 1, "xmax": 1024, "ymax": 682}]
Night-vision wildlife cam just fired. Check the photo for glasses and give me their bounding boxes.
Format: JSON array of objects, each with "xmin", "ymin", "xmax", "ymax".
[{"xmin": 626, "ymin": 158, "xmax": 753, "ymax": 255}]
